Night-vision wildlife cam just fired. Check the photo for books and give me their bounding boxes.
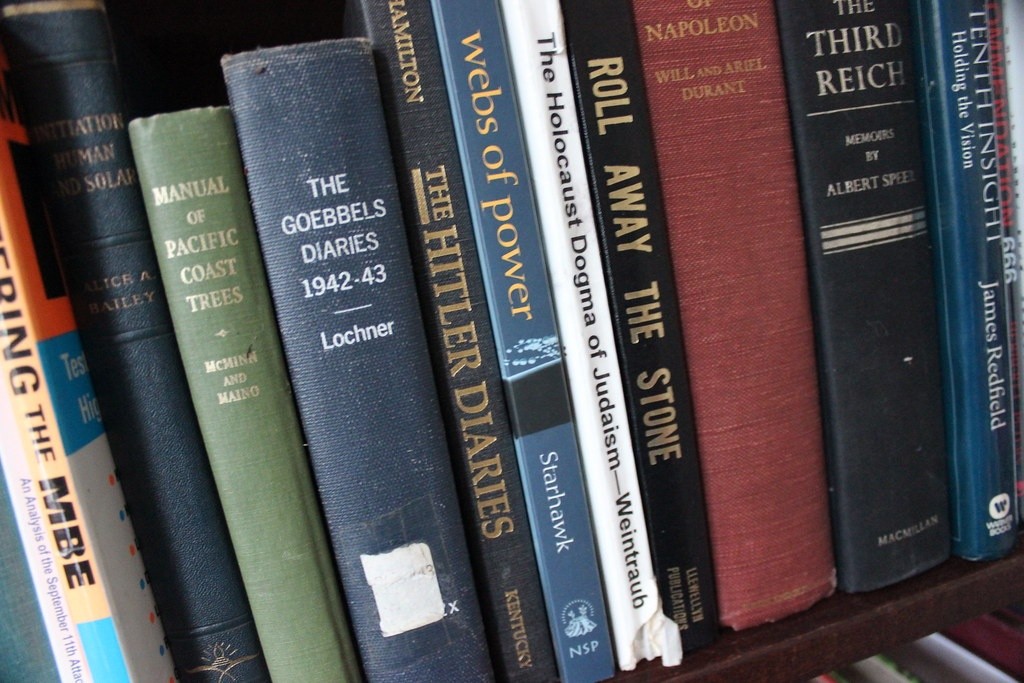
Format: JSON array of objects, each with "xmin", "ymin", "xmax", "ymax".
[{"xmin": 0, "ymin": 0, "xmax": 1024, "ymax": 683}]
